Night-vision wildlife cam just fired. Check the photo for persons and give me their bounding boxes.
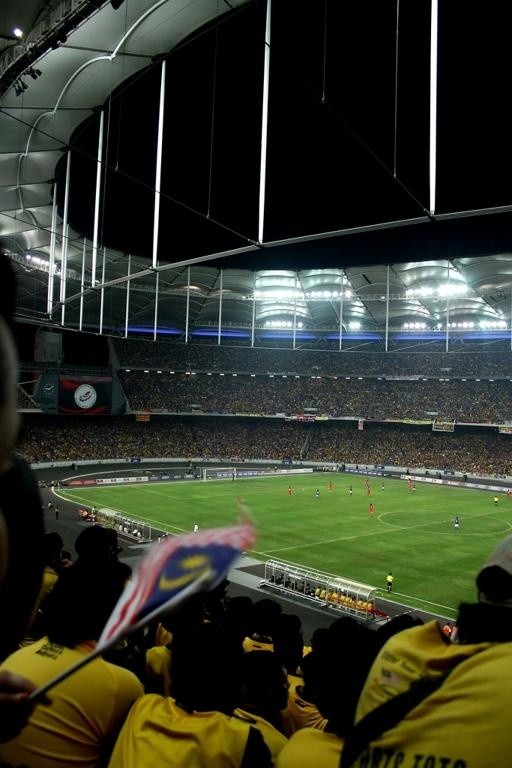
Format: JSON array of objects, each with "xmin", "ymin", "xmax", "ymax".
[
  {"xmin": 0, "ymin": 504, "xmax": 512, "ymax": 768},
  {"xmin": 15, "ymin": 330, "xmax": 512, "ymax": 497},
  {"xmin": 0, "ymin": 322, "xmax": 45, "ymax": 662},
  {"xmin": 193, "ymin": 524, "xmax": 200, "ymax": 533},
  {"xmin": 369, "ymin": 504, "xmax": 375, "ymax": 513},
  {"xmin": 387, "ymin": 573, "xmax": 393, "ymax": 593},
  {"xmin": 454, "ymin": 516, "xmax": 459, "ymax": 530},
  {"xmin": 493, "ymin": 496, "xmax": 498, "ymax": 508}
]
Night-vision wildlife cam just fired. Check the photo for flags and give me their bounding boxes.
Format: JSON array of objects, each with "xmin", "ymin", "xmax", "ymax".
[{"xmin": 93, "ymin": 524, "xmax": 255, "ymax": 655}]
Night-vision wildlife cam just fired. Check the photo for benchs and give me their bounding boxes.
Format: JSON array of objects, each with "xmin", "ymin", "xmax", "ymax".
[
  {"xmin": 256, "ymin": 577, "xmax": 375, "ymax": 625},
  {"xmin": 114, "ymin": 529, "xmax": 152, "ymax": 544}
]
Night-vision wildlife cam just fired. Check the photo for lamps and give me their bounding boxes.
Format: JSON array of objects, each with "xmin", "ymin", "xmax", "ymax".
[{"xmin": 12, "ymin": 28, "xmax": 76, "ymax": 96}]
